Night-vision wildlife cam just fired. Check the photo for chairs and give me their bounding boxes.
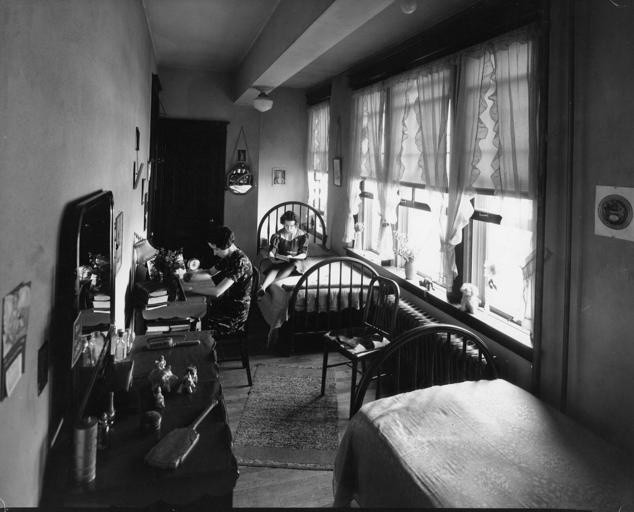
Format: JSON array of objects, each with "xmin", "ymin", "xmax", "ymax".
[
  {"xmin": 321, "ymin": 276, "xmax": 400, "ymax": 420},
  {"xmin": 214, "ymin": 266, "xmax": 260, "ymax": 385}
]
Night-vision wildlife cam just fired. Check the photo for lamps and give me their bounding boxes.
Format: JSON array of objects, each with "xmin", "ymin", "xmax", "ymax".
[
  {"xmin": 252, "ymin": 85, "xmax": 274, "ymax": 113},
  {"xmin": 133, "ymin": 238, "xmax": 159, "ymax": 264}
]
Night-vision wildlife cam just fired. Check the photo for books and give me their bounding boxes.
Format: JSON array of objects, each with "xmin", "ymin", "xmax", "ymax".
[
  {"xmin": 92, "ymin": 301, "xmax": 111, "ymax": 309},
  {"xmin": 94, "ymin": 293, "xmax": 111, "ymax": 301},
  {"xmin": 93, "ymin": 308, "xmax": 110, "ymax": 314},
  {"xmin": 133, "ymin": 280, "xmax": 190, "ymax": 337}
]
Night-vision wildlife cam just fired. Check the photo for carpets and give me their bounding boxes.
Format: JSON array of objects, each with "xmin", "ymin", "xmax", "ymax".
[{"xmin": 230, "ymin": 364, "xmax": 338, "ymax": 467}]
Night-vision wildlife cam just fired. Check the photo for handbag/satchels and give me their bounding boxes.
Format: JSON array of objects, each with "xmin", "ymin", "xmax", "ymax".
[{"xmin": 226, "ymin": 168, "xmax": 252, "ymax": 195}]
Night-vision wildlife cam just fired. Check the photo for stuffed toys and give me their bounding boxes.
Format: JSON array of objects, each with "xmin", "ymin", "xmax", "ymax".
[{"xmin": 459, "ymin": 282, "xmax": 479, "ymax": 314}]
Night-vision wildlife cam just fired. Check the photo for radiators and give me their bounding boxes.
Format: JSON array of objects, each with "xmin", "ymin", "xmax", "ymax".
[{"xmin": 377, "ymin": 294, "xmax": 496, "ymax": 392}]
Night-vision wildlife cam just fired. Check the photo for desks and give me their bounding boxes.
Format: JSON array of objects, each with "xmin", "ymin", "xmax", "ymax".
[
  {"xmin": 134, "ymin": 268, "xmax": 216, "ymax": 331},
  {"xmin": 39, "ymin": 329, "xmax": 239, "ymax": 511}
]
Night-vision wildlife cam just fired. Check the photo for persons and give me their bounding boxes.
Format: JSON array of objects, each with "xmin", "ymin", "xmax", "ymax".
[
  {"xmin": 256, "ymin": 211, "xmax": 308, "ymax": 300},
  {"xmin": 183, "ymin": 225, "xmax": 254, "ymax": 339}
]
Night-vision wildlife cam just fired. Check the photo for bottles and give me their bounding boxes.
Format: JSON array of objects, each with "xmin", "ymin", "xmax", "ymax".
[
  {"xmin": 81, "ymin": 330, "xmax": 106, "ymax": 368},
  {"xmin": 111, "ymin": 328, "xmax": 134, "ymax": 365},
  {"xmin": 70, "ymin": 413, "xmax": 111, "ymax": 484},
  {"xmin": 354, "ymin": 232, "xmax": 363, "ymax": 253},
  {"xmin": 405, "ymin": 256, "xmax": 418, "ymax": 280}
]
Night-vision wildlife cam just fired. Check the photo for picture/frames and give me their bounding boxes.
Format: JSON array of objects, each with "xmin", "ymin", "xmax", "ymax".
[
  {"xmin": 272, "ymin": 167, "xmax": 287, "ymax": 186},
  {"xmin": 333, "ymin": 158, "xmax": 342, "ymax": 188},
  {"xmin": 134, "ymin": 127, "xmax": 151, "ymax": 230}
]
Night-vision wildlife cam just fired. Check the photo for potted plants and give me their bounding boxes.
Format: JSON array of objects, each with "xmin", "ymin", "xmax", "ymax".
[{"xmin": 151, "ymin": 248, "xmax": 185, "ymax": 284}]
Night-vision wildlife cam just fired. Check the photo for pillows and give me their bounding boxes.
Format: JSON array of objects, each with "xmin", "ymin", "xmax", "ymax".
[{"xmin": 261, "ymin": 243, "xmax": 328, "ymax": 258}]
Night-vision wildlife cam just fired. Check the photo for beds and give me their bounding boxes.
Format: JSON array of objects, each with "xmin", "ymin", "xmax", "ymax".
[
  {"xmin": 332, "ymin": 324, "xmax": 634, "ymax": 512},
  {"xmin": 256, "ymin": 201, "xmax": 381, "ymax": 354}
]
